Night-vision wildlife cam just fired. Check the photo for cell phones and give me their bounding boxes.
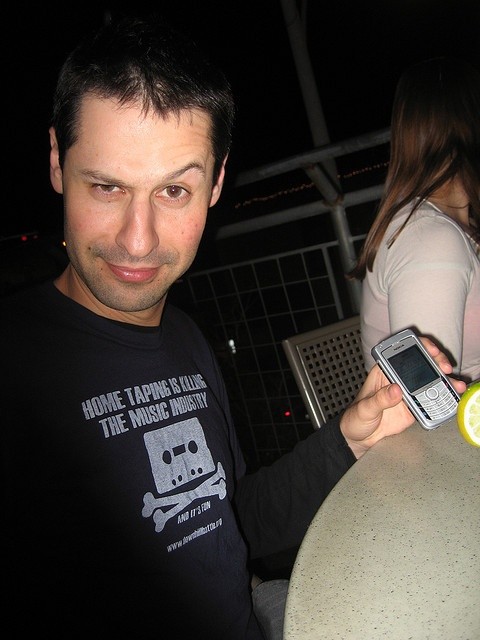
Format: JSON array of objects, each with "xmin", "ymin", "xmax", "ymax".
[{"xmin": 370, "ymin": 331, "xmax": 461, "ymax": 431}]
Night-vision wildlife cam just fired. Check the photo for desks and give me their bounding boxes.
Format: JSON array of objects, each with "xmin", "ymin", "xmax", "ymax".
[{"xmin": 280, "ymin": 415, "xmax": 480, "ymax": 639}]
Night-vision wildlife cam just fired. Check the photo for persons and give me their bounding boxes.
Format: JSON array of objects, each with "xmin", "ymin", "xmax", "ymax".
[
  {"xmin": 0, "ymin": 26, "xmax": 466, "ymax": 640},
  {"xmin": 345, "ymin": 65, "xmax": 480, "ymax": 400}
]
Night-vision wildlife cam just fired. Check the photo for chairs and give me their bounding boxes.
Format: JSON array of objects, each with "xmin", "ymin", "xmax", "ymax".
[{"xmin": 276, "ymin": 312, "xmax": 370, "ymax": 428}]
